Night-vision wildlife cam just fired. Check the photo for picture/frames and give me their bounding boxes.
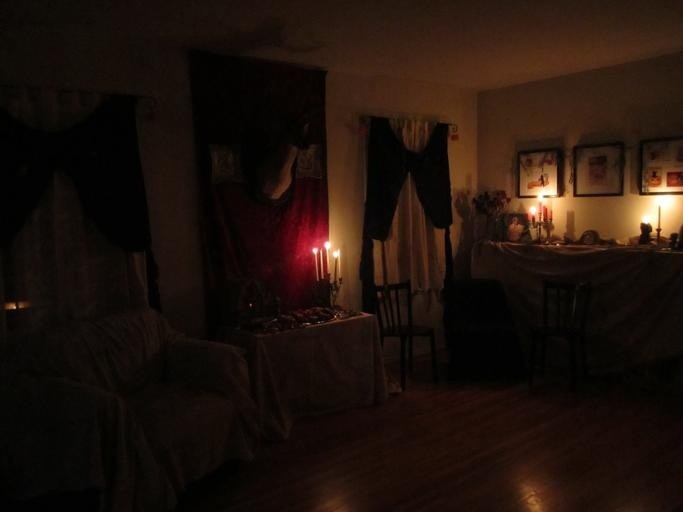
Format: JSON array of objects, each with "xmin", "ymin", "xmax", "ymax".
[{"xmin": 517, "ymin": 137, "xmax": 682, "ymax": 199}]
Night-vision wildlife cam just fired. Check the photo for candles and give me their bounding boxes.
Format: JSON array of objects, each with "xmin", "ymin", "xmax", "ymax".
[{"xmin": 312, "ymin": 238, "xmax": 343, "ymax": 284}]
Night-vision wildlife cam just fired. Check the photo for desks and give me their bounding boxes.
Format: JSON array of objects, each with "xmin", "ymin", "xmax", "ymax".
[
  {"xmin": 216, "ymin": 309, "xmax": 378, "ymax": 422},
  {"xmin": 470, "ymin": 239, "xmax": 683, "ymax": 396}
]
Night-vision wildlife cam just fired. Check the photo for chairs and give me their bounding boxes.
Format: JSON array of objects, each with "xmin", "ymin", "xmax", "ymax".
[
  {"xmin": 364, "ymin": 278, "xmax": 437, "ymax": 392},
  {"xmin": 527, "ymin": 277, "xmax": 591, "ymax": 393},
  {"xmin": 16, "ymin": 305, "xmax": 251, "ymax": 510}
]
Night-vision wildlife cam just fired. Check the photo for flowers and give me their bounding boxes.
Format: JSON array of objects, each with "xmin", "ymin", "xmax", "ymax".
[{"xmin": 474, "ymin": 190, "xmax": 510, "ymax": 222}]
